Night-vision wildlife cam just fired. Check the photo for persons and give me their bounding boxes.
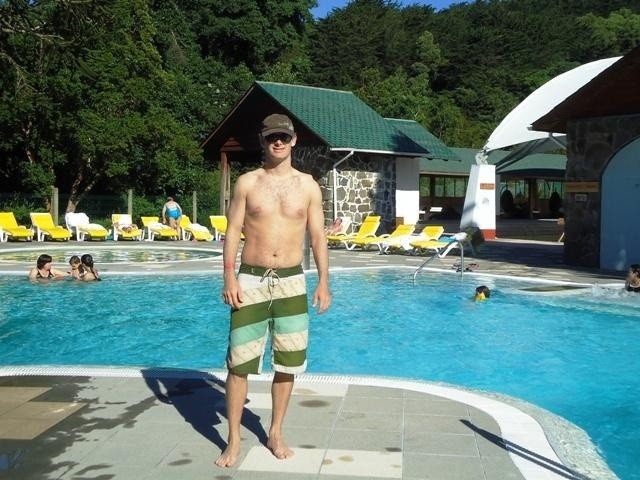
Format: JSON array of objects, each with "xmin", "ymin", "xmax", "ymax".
[
  {"xmin": 625, "ymin": 263, "xmax": 640, "ymax": 292},
  {"xmin": 112, "ymin": 223, "xmax": 136, "ymax": 232},
  {"xmin": 213, "ymin": 112, "xmax": 331, "ymax": 467},
  {"xmin": 162, "ymin": 197, "xmax": 182, "ymax": 241},
  {"xmin": 72, "ymin": 254, "xmax": 98, "ymax": 282},
  {"xmin": 69, "ymin": 256, "xmax": 83, "ymax": 276},
  {"xmin": 323, "ymin": 217, "xmax": 342, "ymax": 235},
  {"xmin": 475, "ymin": 286, "xmax": 489, "ymax": 300},
  {"xmin": 28, "ymin": 254, "xmax": 55, "ymax": 282}
]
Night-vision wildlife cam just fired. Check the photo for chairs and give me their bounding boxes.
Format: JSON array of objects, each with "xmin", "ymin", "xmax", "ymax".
[
  {"xmin": 1, "ymin": 210, "xmax": 34, "ymax": 242},
  {"xmin": 208, "ymin": 214, "xmax": 246, "ymax": 243},
  {"xmin": 138, "ymin": 214, "xmax": 179, "ymax": 242},
  {"xmin": 28, "ymin": 211, "xmax": 72, "ymax": 244},
  {"xmin": 110, "ymin": 213, "xmax": 143, "ymax": 242},
  {"xmin": 177, "ymin": 213, "xmax": 215, "ymax": 242},
  {"xmin": 63, "ymin": 212, "xmax": 108, "ymax": 242},
  {"xmin": 322, "ymin": 215, "xmax": 480, "ymax": 259}
]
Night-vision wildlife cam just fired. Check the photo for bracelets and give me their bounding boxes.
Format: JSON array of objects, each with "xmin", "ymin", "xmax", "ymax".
[{"xmin": 222, "ymin": 262, "xmax": 236, "ymax": 269}]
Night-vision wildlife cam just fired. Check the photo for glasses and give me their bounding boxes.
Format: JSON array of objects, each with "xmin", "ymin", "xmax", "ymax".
[{"xmin": 264, "ymin": 133, "xmax": 294, "ymax": 144}]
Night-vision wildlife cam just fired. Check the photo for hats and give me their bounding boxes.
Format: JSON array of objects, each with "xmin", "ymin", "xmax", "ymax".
[{"xmin": 261, "ymin": 113, "xmax": 295, "ymax": 137}]
